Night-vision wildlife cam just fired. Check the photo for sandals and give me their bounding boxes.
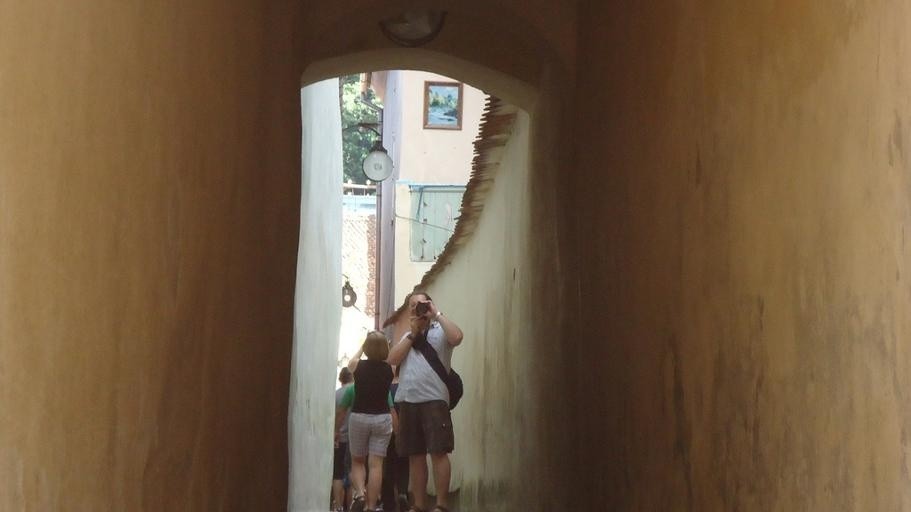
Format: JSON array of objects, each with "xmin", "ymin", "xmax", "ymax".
[{"xmin": 407, "ymin": 504, "xmax": 448, "ymax": 512}]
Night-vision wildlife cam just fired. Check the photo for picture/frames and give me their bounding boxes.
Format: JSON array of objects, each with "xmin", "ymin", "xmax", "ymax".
[{"xmin": 423, "ymin": 79, "xmax": 464, "ymax": 131}]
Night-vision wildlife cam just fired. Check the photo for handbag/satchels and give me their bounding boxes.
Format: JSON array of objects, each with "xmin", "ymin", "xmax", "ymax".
[{"xmin": 449, "ymin": 367, "xmax": 463, "ymax": 410}]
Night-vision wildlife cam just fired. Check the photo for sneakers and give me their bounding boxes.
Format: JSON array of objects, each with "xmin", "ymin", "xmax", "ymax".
[{"xmin": 330, "ymin": 492, "xmax": 410, "ymax": 512}]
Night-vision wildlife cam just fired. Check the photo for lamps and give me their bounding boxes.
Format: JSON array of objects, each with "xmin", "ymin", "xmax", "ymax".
[{"xmin": 357, "ymin": 122, "xmax": 393, "ymax": 182}]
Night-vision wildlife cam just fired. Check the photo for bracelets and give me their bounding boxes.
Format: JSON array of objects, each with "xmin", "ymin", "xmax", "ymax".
[{"xmin": 435, "ymin": 311, "xmax": 444, "ymax": 320}]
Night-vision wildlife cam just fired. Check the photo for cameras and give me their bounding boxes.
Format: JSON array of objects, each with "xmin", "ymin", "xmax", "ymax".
[{"xmin": 416, "ymin": 304, "xmax": 429, "ymax": 317}]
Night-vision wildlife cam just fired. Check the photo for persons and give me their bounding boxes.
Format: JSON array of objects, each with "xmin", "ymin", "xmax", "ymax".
[
  {"xmin": 331, "ymin": 364, "xmax": 411, "ymax": 512},
  {"xmin": 347, "ymin": 328, "xmax": 394, "ymax": 511},
  {"xmin": 385, "ymin": 290, "xmax": 465, "ymax": 511}
]
